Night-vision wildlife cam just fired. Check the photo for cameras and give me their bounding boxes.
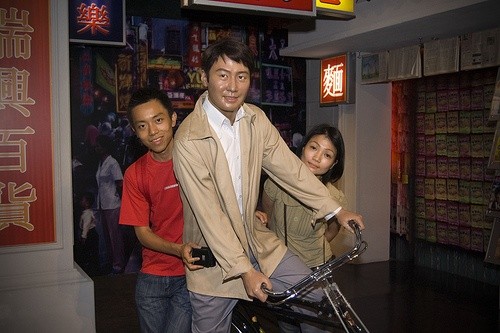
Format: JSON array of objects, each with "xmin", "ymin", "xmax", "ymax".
[{"xmin": 192, "ymin": 247, "xmax": 216, "ymax": 269}]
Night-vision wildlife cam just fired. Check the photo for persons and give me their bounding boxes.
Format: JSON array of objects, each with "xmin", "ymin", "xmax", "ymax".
[
  {"xmin": 262, "ymin": 123, "xmax": 346, "ymax": 333},
  {"xmin": 119, "ymin": 86, "xmax": 267, "ymax": 333},
  {"xmin": 71, "ymin": 132, "xmax": 128, "ymax": 275},
  {"xmin": 172, "ymin": 39, "xmax": 365, "ymax": 333}
]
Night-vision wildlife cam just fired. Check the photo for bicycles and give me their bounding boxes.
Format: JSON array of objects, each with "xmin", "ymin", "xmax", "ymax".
[{"xmin": 228, "ymin": 218, "xmax": 376, "ymax": 331}]
